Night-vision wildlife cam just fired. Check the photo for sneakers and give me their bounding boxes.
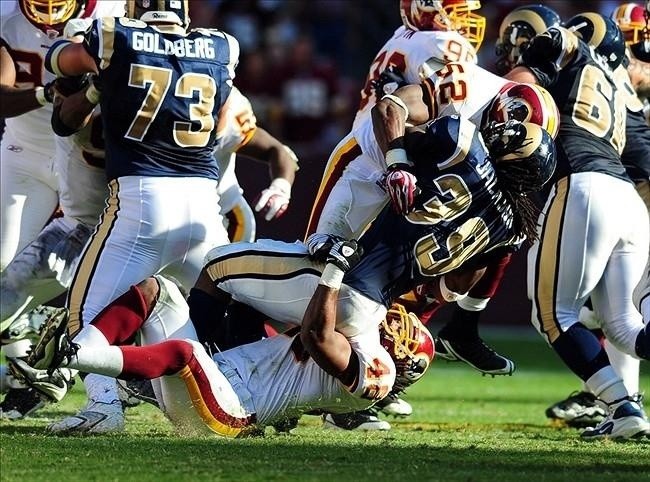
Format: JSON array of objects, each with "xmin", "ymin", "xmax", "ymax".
[
  {"xmin": 45, "ymin": 398, "xmax": 125, "ymax": 433},
  {"xmin": 1, "ymin": 304, "xmax": 82, "ymax": 419},
  {"xmin": 433, "ymin": 329, "xmax": 515, "ymax": 376},
  {"xmin": 546, "ymin": 390, "xmax": 650, "ymax": 440},
  {"xmin": 333, "ymin": 413, "xmax": 390, "ymax": 431},
  {"xmin": 380, "ymin": 394, "xmax": 412, "ymax": 416}
]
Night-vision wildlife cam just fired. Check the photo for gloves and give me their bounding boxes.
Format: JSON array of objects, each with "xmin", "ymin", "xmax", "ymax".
[
  {"xmin": 44, "ymin": 71, "xmax": 92, "ymax": 103},
  {"xmin": 256, "ymin": 178, "xmax": 292, "ymax": 221},
  {"xmin": 387, "ymin": 167, "xmax": 418, "ymax": 215},
  {"xmin": 307, "ymin": 232, "xmax": 364, "ymax": 274}
]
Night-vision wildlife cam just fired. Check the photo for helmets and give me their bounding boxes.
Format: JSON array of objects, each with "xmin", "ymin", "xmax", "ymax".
[
  {"xmin": 24, "ymin": 0, "xmax": 83, "ymax": 25},
  {"xmin": 495, "ymin": 3, "xmax": 650, "ymax": 71},
  {"xmin": 400, "ymin": 0, "xmax": 486, "ymax": 54},
  {"xmin": 482, "ymin": 82, "xmax": 561, "ymax": 195},
  {"xmin": 379, "ymin": 302, "xmax": 435, "ymax": 396},
  {"xmin": 125, "ymin": 0, "xmax": 190, "ymax": 29}
]
